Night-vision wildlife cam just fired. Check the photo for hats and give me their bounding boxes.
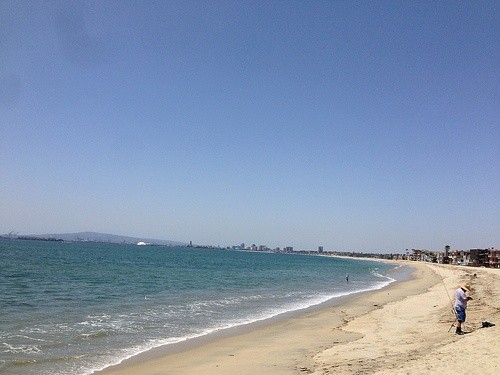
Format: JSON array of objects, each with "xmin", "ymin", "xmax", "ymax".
[{"xmin": 461, "ymin": 283, "xmax": 471, "ymax": 292}]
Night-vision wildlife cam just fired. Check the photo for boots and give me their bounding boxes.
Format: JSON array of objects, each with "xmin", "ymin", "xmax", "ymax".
[{"xmin": 456, "ymin": 326, "xmax": 463, "ymax": 335}]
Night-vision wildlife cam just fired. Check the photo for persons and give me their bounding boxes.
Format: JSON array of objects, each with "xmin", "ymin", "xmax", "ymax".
[
  {"xmin": 454, "ymin": 283, "xmax": 473, "ymax": 335},
  {"xmin": 344, "ymin": 273, "xmax": 349, "ymax": 281}
]
